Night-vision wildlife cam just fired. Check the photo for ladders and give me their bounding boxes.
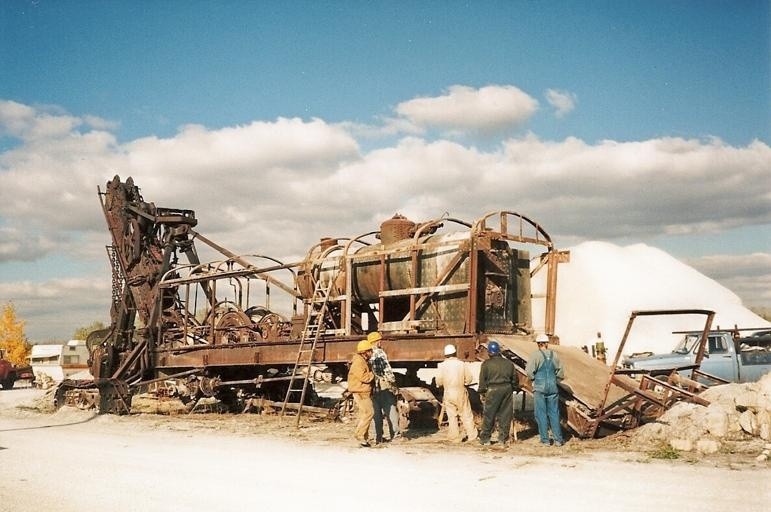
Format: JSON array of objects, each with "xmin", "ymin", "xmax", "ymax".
[{"xmin": 280, "ymin": 280, "xmax": 333, "ymax": 430}]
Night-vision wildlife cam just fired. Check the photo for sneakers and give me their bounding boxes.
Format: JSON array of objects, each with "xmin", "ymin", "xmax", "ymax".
[
  {"xmin": 477, "ymin": 441, "xmax": 491, "ymax": 446},
  {"xmin": 540, "ymin": 442, "xmax": 551, "ymax": 447},
  {"xmin": 352, "ymin": 433, "xmax": 368, "ymax": 445},
  {"xmin": 493, "ymin": 441, "xmax": 505, "ymax": 447},
  {"xmin": 555, "ymin": 441, "xmax": 562, "ymax": 447},
  {"xmin": 376, "ymin": 438, "xmax": 392, "ymax": 445}
]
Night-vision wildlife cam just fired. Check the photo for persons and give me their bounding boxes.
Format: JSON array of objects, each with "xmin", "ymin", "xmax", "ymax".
[
  {"xmin": 431, "ymin": 343, "xmax": 478, "ymax": 445},
  {"xmin": 474, "ymin": 340, "xmax": 521, "ymax": 447},
  {"xmin": 345, "ymin": 340, "xmax": 375, "ymax": 447},
  {"xmin": 523, "ymin": 333, "xmax": 567, "ymax": 449},
  {"xmin": 366, "ymin": 331, "xmax": 403, "ymax": 443}
]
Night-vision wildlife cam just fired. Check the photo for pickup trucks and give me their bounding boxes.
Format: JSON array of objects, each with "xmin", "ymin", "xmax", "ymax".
[{"xmin": 621, "ymin": 331, "xmax": 771, "ymax": 387}]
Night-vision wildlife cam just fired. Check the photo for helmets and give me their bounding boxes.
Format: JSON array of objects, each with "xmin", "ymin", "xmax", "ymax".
[
  {"xmin": 368, "ymin": 331, "xmax": 383, "ymax": 343},
  {"xmin": 444, "ymin": 344, "xmax": 456, "ymax": 356},
  {"xmin": 536, "ymin": 334, "xmax": 550, "ymax": 342},
  {"xmin": 357, "ymin": 339, "xmax": 373, "ymax": 352},
  {"xmin": 487, "ymin": 342, "xmax": 500, "ymax": 353}
]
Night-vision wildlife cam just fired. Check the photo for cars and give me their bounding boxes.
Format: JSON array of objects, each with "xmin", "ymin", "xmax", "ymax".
[{"xmin": 0, "ymin": 349, "xmax": 35, "ymax": 389}]
